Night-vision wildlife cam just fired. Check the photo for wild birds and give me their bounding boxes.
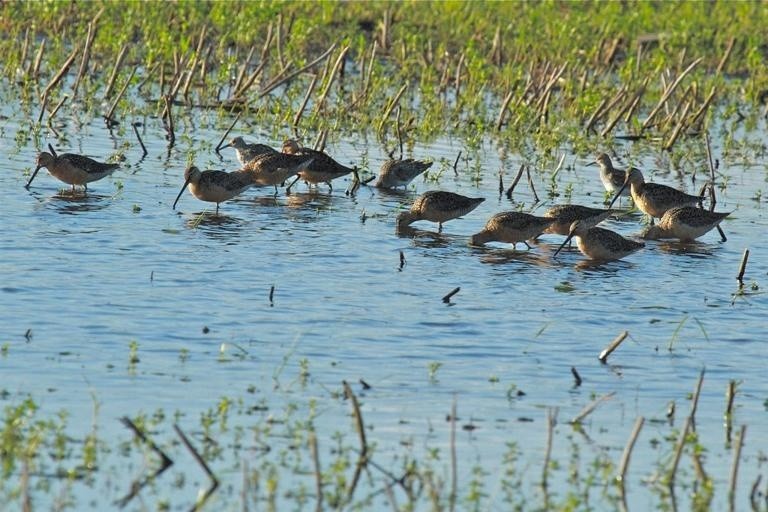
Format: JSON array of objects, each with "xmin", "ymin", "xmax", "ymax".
[
  {"xmin": 640, "ymin": 206, "xmax": 731, "ymax": 243},
  {"xmin": 585, "ymin": 153, "xmax": 630, "ymax": 196},
  {"xmin": 533, "ymin": 204, "xmax": 620, "ymax": 243},
  {"xmin": 281, "ymin": 140, "xmax": 362, "ymax": 194},
  {"xmin": 25, "ymin": 152, "xmax": 120, "ymax": 193},
  {"xmin": 472, "ymin": 212, "xmax": 564, "ymax": 250},
  {"xmin": 609, "ymin": 167, "xmax": 707, "ymax": 225},
  {"xmin": 373, "ymin": 159, "xmax": 433, "ymax": 189},
  {"xmin": 236, "ymin": 152, "xmax": 314, "ymax": 195},
  {"xmin": 173, "ymin": 167, "xmax": 255, "ymax": 209},
  {"xmin": 553, "ymin": 219, "xmax": 646, "ymax": 267},
  {"xmin": 396, "ymin": 191, "xmax": 485, "ymax": 233},
  {"xmin": 217, "ymin": 136, "xmax": 279, "ymax": 166}
]
